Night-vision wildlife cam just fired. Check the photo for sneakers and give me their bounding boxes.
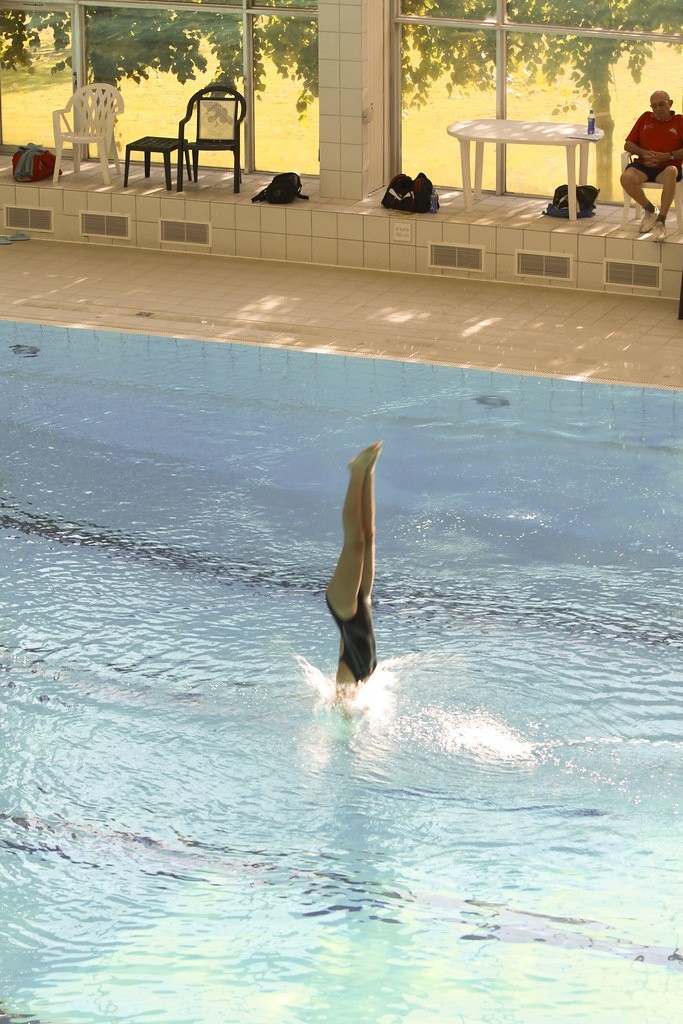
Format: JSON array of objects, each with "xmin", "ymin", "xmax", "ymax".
[
  {"xmin": 653, "ymin": 221, "xmax": 666, "ymax": 242},
  {"xmin": 639, "ymin": 204, "xmax": 660, "ymax": 232}
]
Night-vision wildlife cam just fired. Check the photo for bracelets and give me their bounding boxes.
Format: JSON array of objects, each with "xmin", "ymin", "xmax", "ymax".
[{"xmin": 669, "ymin": 151, "xmax": 673, "ymax": 160}]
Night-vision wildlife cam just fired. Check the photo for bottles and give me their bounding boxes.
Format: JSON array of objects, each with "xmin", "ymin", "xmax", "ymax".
[
  {"xmin": 430, "ymin": 185, "xmax": 439, "ymax": 213},
  {"xmin": 587, "ymin": 110, "xmax": 595, "ymax": 134}
]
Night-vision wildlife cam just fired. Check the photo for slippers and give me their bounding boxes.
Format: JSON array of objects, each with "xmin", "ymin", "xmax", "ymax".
[
  {"xmin": 0, "ymin": 235, "xmax": 12, "ymax": 244},
  {"xmin": 7, "ymin": 232, "xmax": 31, "ymax": 240}
]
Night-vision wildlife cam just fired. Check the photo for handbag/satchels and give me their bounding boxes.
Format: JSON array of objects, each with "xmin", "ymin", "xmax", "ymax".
[
  {"xmin": 12, "ymin": 146, "xmax": 63, "ymax": 181},
  {"xmin": 552, "ymin": 185, "xmax": 600, "ymax": 210},
  {"xmin": 252, "ymin": 173, "xmax": 309, "ymax": 204}
]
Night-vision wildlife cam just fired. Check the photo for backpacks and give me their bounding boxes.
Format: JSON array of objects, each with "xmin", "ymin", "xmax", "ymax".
[{"xmin": 381, "ymin": 173, "xmax": 439, "ymax": 213}]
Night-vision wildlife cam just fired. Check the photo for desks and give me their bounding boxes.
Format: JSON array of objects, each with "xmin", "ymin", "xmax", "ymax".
[
  {"xmin": 446, "ymin": 119, "xmax": 606, "ymax": 221},
  {"xmin": 125, "ymin": 136, "xmax": 192, "ymax": 191}
]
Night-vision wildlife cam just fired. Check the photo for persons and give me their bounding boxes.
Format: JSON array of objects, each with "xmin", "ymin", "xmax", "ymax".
[
  {"xmin": 325, "ymin": 442, "xmax": 382, "ymax": 695},
  {"xmin": 620, "ymin": 91, "xmax": 683, "ymax": 241}
]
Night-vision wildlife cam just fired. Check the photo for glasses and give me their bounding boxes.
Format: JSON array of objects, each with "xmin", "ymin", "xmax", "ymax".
[{"xmin": 650, "ymin": 101, "xmax": 669, "ymax": 108}]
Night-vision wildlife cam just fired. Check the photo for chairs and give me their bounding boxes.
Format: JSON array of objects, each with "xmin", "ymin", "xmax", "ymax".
[
  {"xmin": 620, "ymin": 151, "xmax": 683, "ymax": 232},
  {"xmin": 52, "ymin": 83, "xmax": 124, "ymax": 185},
  {"xmin": 177, "ymin": 85, "xmax": 247, "ymax": 194}
]
